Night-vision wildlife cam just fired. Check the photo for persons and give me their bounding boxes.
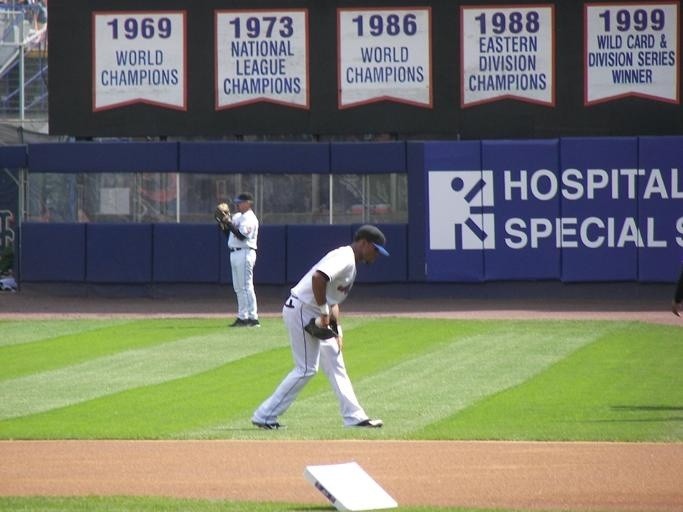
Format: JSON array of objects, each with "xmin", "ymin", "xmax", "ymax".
[
  {"xmin": 251, "ymin": 226, "xmax": 391, "ymax": 429},
  {"xmin": 0, "ymin": 0, "xmax": 47, "ymax": 23},
  {"xmin": 214, "ymin": 192, "xmax": 259, "ymax": 328},
  {"xmin": 671, "ymin": 267, "xmax": 683, "ymax": 318}
]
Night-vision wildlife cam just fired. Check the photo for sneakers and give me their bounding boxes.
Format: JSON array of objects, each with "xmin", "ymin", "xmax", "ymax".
[
  {"xmin": 252, "ymin": 420, "xmax": 287, "ymax": 431},
  {"xmin": 358, "ymin": 418, "xmax": 383, "ymax": 428},
  {"xmin": 228, "ymin": 318, "xmax": 260, "ymax": 329}
]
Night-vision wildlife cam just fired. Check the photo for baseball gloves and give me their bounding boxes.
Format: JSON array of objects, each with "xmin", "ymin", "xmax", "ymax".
[
  {"xmin": 304, "ymin": 313, "xmax": 339, "ymax": 340},
  {"xmin": 215, "ymin": 202, "xmax": 231, "ymax": 230}
]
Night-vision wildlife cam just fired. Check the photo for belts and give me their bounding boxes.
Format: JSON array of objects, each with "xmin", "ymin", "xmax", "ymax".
[{"xmin": 229, "ymin": 246, "xmax": 257, "ymax": 252}]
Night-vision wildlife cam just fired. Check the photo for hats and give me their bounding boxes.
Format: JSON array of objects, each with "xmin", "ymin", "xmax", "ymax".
[
  {"xmin": 234, "ymin": 192, "xmax": 254, "ymax": 203},
  {"xmin": 357, "ymin": 225, "xmax": 390, "ymax": 257}
]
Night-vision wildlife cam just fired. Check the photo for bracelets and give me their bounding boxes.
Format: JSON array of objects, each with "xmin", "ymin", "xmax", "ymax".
[
  {"xmin": 337, "ymin": 325, "xmax": 344, "ymax": 338},
  {"xmin": 316, "ymin": 301, "xmax": 330, "ymax": 317}
]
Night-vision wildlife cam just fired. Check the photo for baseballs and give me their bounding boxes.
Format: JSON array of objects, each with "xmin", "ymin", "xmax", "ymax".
[{"xmin": 315, "ymin": 316, "xmax": 323, "ymax": 327}]
